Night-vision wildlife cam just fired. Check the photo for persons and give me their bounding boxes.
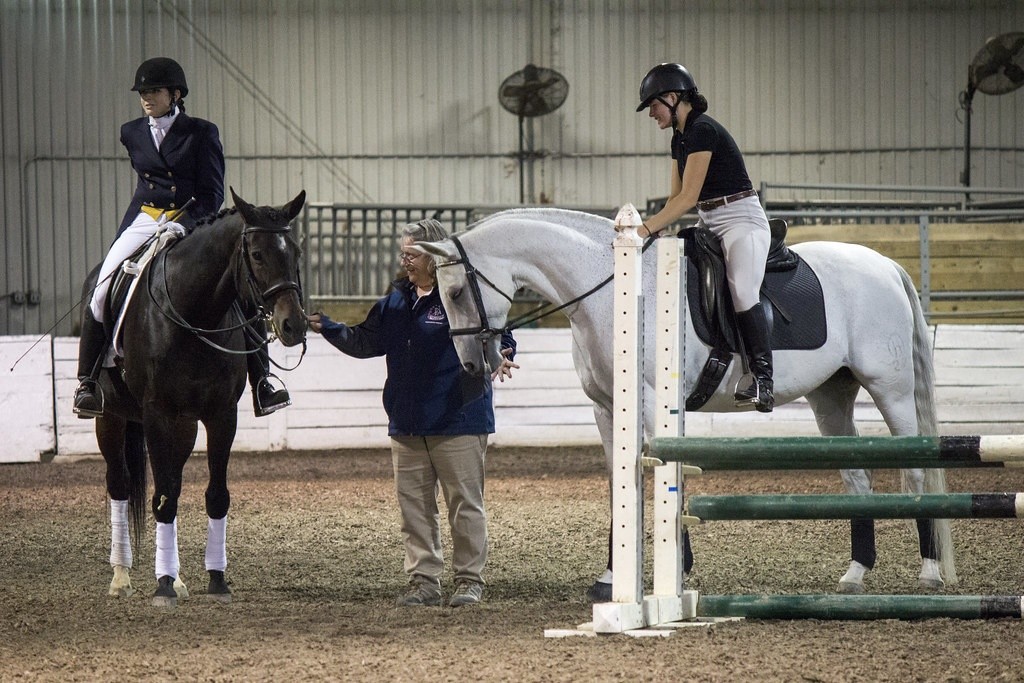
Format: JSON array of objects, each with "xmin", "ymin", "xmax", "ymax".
[
  {"xmin": 614, "ymin": 62, "xmax": 775, "ymax": 413},
  {"xmin": 306, "ymin": 219, "xmax": 520, "ymax": 607},
  {"xmin": 75, "ymin": 57, "xmax": 289, "ymax": 419}
]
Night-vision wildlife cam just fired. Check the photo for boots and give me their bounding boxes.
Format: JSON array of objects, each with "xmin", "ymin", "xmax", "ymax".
[
  {"xmin": 73, "ymin": 304, "xmax": 115, "ymax": 419},
  {"xmin": 734, "ymin": 302, "xmax": 774, "ymax": 413},
  {"xmin": 243, "ymin": 308, "xmax": 289, "ymax": 418}
]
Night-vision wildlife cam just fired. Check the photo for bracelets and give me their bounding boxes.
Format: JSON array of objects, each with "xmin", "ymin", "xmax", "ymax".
[{"xmin": 643, "ymin": 222, "xmax": 652, "ymax": 236}]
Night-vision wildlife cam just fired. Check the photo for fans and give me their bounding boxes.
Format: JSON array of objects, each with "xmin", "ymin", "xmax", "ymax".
[
  {"xmin": 498, "ymin": 63, "xmax": 568, "ymax": 205},
  {"xmin": 962, "ymin": 31, "xmax": 1024, "ymax": 204}
]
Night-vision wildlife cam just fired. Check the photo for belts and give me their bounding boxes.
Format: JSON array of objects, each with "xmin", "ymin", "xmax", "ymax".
[{"xmin": 695, "ymin": 190, "xmax": 756, "ymax": 212}]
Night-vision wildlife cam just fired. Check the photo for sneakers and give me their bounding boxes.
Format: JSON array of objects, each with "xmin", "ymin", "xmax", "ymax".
[
  {"xmin": 396, "ymin": 580, "xmax": 444, "ymax": 607},
  {"xmin": 448, "ymin": 579, "xmax": 482, "ymax": 607}
]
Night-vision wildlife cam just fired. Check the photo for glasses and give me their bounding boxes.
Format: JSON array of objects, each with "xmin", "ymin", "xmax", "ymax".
[{"xmin": 399, "ymin": 252, "xmax": 423, "ymax": 264}]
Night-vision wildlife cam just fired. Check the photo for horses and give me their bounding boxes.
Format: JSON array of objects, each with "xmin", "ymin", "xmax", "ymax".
[
  {"xmin": 402, "ymin": 208, "xmax": 961, "ymax": 594},
  {"xmin": 77, "ymin": 185, "xmax": 308, "ymax": 610}
]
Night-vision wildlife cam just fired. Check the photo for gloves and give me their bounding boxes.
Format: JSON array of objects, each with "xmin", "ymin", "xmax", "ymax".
[{"xmin": 157, "ymin": 220, "xmax": 186, "ymax": 237}]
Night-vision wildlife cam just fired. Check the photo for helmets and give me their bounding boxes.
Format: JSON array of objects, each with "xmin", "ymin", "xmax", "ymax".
[
  {"xmin": 130, "ymin": 57, "xmax": 189, "ymax": 98},
  {"xmin": 636, "ymin": 62, "xmax": 697, "ymax": 112}
]
